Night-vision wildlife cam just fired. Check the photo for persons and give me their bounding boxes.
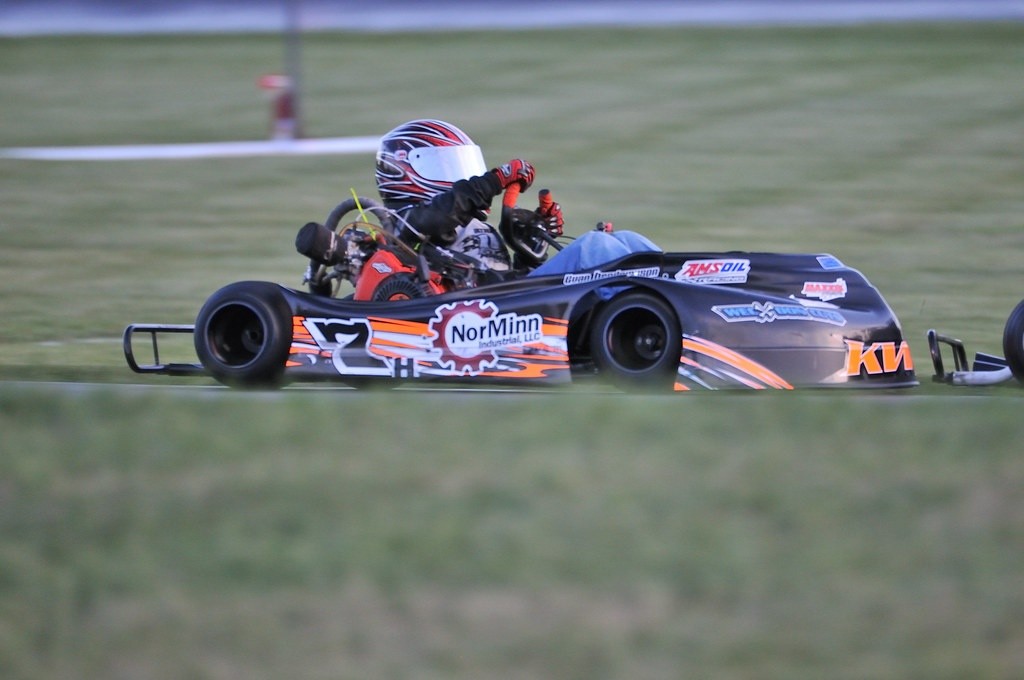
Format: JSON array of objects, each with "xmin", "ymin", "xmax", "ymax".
[{"xmin": 354, "ymin": 118, "xmax": 663, "ymax": 301}]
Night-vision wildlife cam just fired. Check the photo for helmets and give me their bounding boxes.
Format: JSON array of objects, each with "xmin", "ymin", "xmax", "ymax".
[{"xmin": 374, "ymin": 118, "xmax": 492, "ymax": 222}]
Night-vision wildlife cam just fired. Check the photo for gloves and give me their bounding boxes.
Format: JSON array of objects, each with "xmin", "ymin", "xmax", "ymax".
[
  {"xmin": 495, "ymin": 158, "xmax": 536, "ymax": 193},
  {"xmin": 534, "ymin": 202, "xmax": 565, "ymax": 239}
]
